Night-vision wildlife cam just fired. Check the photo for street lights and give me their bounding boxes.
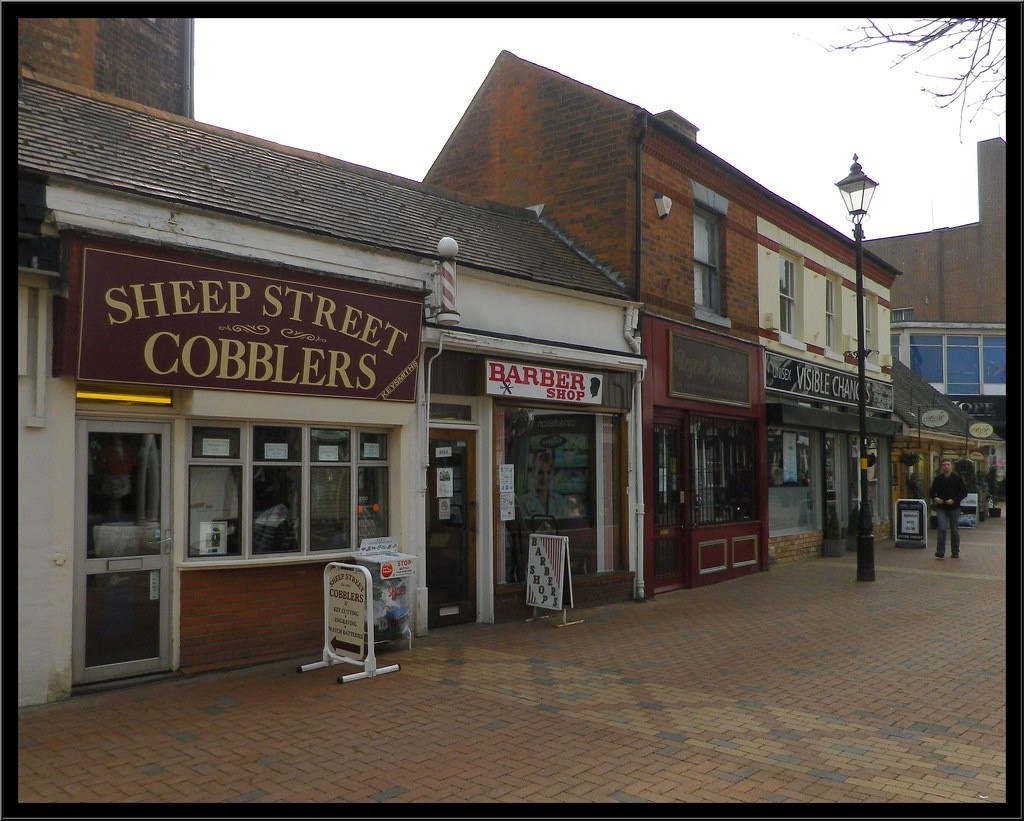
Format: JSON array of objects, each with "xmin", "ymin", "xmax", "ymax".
[{"xmin": 835, "ymin": 152, "xmax": 881, "ymax": 582}]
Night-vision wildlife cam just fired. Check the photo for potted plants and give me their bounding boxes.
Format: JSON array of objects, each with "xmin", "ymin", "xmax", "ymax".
[
  {"xmin": 898, "ymin": 450, "xmax": 923, "ymax": 479},
  {"xmin": 985, "ymin": 464, "xmax": 1002, "ymax": 518},
  {"xmin": 822, "ymin": 500, "xmax": 860, "ymax": 557}
]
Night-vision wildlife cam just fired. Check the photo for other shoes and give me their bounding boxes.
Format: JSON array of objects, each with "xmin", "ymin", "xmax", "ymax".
[
  {"xmin": 951, "ymin": 553, "xmax": 959, "ymax": 558},
  {"xmin": 935, "ymin": 553, "xmax": 945, "ymax": 560}
]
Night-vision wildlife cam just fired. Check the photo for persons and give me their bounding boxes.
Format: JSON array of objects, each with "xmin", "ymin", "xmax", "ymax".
[
  {"xmin": 518, "ymin": 451, "xmax": 569, "ymax": 576},
  {"xmin": 929, "ymin": 457, "xmax": 968, "ymax": 558},
  {"xmin": 99, "ymin": 433, "xmax": 136, "ymax": 521}
]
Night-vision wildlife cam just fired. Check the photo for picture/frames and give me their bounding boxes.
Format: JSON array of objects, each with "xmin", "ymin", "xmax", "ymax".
[{"xmin": 198, "ymin": 521, "xmax": 228, "ymax": 556}]
